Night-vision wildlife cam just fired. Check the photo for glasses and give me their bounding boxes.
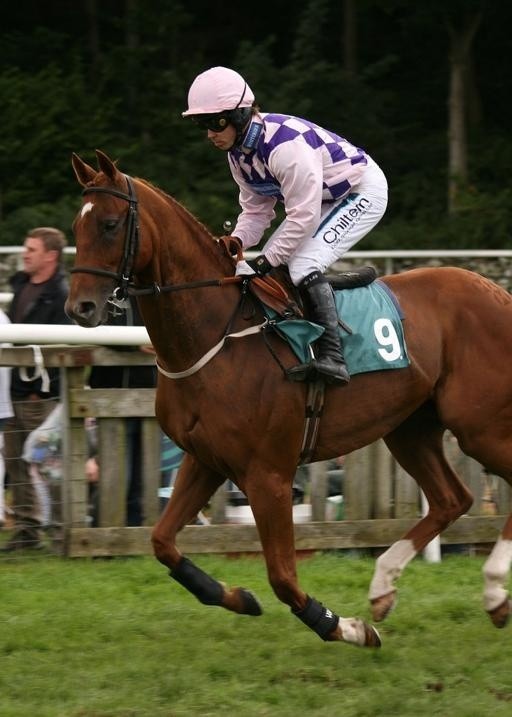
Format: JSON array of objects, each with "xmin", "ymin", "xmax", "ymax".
[{"xmin": 199, "ymin": 111, "xmax": 233, "ymax": 132}]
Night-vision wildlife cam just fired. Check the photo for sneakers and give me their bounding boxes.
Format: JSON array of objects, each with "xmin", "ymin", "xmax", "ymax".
[
  {"xmin": 1, "ymin": 540, "xmax": 45, "ymax": 552},
  {"xmin": 46, "ymin": 541, "xmax": 66, "ymax": 556}
]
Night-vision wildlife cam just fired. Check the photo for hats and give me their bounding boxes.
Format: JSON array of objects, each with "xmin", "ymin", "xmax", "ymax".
[{"xmin": 181, "ymin": 66, "xmax": 256, "ymax": 118}]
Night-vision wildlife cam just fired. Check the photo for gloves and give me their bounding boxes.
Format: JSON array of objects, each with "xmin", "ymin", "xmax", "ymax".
[
  {"xmin": 224, "ymin": 237, "xmax": 243, "ymax": 256},
  {"xmin": 251, "ymin": 254, "xmax": 274, "ymax": 277}
]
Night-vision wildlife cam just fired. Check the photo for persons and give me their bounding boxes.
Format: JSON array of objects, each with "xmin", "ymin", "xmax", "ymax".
[
  {"xmin": 181, "ymin": 66, "xmax": 388, "ymax": 385},
  {"xmin": 0, "ymin": 226, "xmax": 157, "ymax": 552}
]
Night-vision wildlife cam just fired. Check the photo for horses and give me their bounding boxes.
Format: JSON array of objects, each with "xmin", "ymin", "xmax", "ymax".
[{"xmin": 64, "ymin": 149, "xmax": 512, "ymax": 649}]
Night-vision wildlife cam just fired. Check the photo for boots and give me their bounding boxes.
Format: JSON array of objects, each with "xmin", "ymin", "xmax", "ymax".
[{"xmin": 286, "ymin": 270, "xmax": 351, "ymax": 385}]
